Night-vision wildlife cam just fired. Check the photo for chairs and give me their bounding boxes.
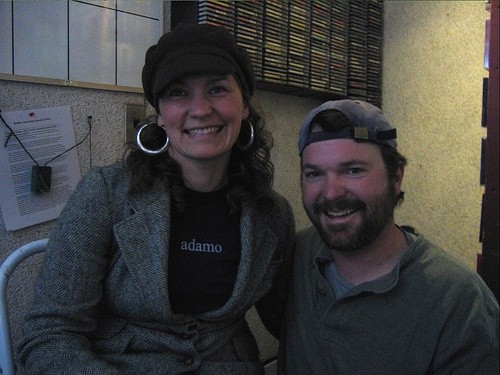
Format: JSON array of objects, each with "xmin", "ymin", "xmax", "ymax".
[{"xmin": 0, "ymin": 237, "xmax": 51, "ymax": 375}]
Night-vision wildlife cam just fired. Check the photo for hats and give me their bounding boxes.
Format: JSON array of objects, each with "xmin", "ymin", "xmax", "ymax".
[
  {"xmin": 142, "ymin": 23, "xmax": 256, "ymax": 113},
  {"xmin": 298, "ymin": 100, "xmax": 396, "ymax": 157}
]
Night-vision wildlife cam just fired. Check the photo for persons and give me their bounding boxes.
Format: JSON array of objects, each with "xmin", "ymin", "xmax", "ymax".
[
  {"xmin": 14, "ymin": 22, "xmax": 298, "ymax": 375},
  {"xmin": 276, "ymin": 98, "xmax": 500, "ymax": 375}
]
